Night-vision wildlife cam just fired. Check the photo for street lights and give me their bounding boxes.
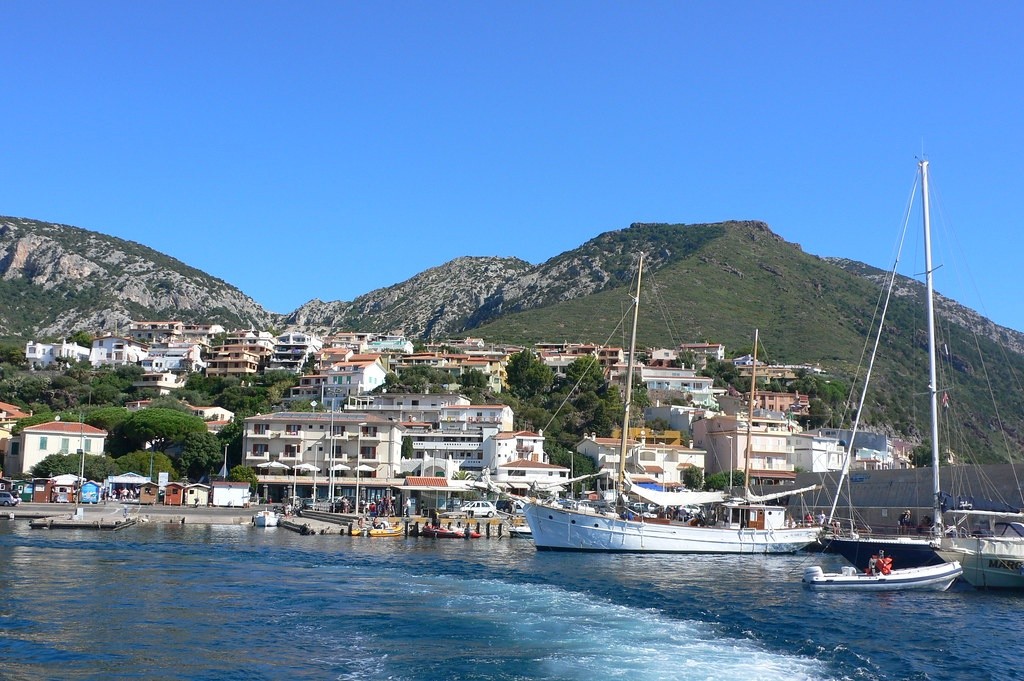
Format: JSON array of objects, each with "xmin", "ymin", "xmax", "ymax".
[
  {"xmin": 74, "ymin": 449, "xmax": 83, "ymax": 513},
  {"xmin": 80, "ymin": 436, "xmax": 89, "ymax": 485},
  {"xmin": 224, "ymin": 443, "xmax": 229, "ymax": 478},
  {"xmin": 314, "ymin": 442, "xmax": 322, "ymax": 501},
  {"xmin": 567, "ymin": 450, "xmax": 574, "ymax": 500},
  {"xmin": 331, "ymin": 433, "xmax": 341, "ymax": 504},
  {"xmin": 356, "ymin": 422, "xmax": 367, "ymax": 513},
  {"xmin": 290, "ymin": 444, "xmax": 297, "ymax": 508}
]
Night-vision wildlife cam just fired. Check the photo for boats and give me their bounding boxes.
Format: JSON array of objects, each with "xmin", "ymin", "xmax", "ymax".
[
  {"xmin": 509, "ymin": 525, "xmax": 533, "ymax": 539},
  {"xmin": 252, "ymin": 508, "xmax": 278, "ymax": 528},
  {"xmin": 421, "ymin": 524, "xmax": 480, "ymax": 538},
  {"xmin": 802, "ymin": 551, "xmax": 966, "ymax": 590},
  {"xmin": 350, "ymin": 520, "xmax": 404, "ymax": 537},
  {"xmin": 930, "ymin": 507, "xmax": 1024, "ymax": 590}
]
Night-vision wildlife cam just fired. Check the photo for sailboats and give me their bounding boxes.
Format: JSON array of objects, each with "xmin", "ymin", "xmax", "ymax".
[
  {"xmin": 813, "ymin": 156, "xmax": 1024, "ymax": 574},
  {"xmin": 494, "ymin": 250, "xmax": 825, "ymax": 555}
]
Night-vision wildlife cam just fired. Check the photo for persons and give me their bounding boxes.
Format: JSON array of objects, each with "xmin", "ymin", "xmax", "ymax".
[
  {"xmin": 341, "ymin": 497, "xmax": 346, "ymax": 508},
  {"xmin": 919, "ymin": 515, "xmax": 931, "ymax": 527},
  {"xmin": 698, "ymin": 511, "xmax": 702, "ymax": 517},
  {"xmin": 884, "ymin": 553, "xmax": 892, "ymax": 570},
  {"xmin": 286, "ymin": 502, "xmax": 291, "ymax": 512},
  {"xmin": 403, "ymin": 497, "xmax": 411, "ymax": 517},
  {"xmin": 507, "ymin": 501, "xmax": 514, "ymax": 521},
  {"xmin": 690, "ymin": 510, "xmax": 694, "ymax": 518},
  {"xmin": 759, "ymin": 406, "xmax": 763, "ymax": 417},
  {"xmin": 369, "ymin": 503, "xmax": 376, "ymax": 512},
  {"xmin": 374, "ymin": 516, "xmax": 379, "ymax": 525},
  {"xmin": 420, "ymin": 500, "xmax": 425, "ymax": 518},
  {"xmin": 930, "ymin": 534, "xmax": 942, "ymax": 550},
  {"xmin": 806, "ymin": 510, "xmax": 825, "ymax": 525}
]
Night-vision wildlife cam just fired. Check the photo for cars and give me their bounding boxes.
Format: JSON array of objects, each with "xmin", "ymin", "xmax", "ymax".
[
  {"xmin": 286, "ymin": 494, "xmax": 368, "ymax": 514},
  {"xmin": 0, "ymin": 490, "xmax": 22, "ymax": 507},
  {"xmin": 459, "ymin": 500, "xmax": 497, "ymax": 517}
]
